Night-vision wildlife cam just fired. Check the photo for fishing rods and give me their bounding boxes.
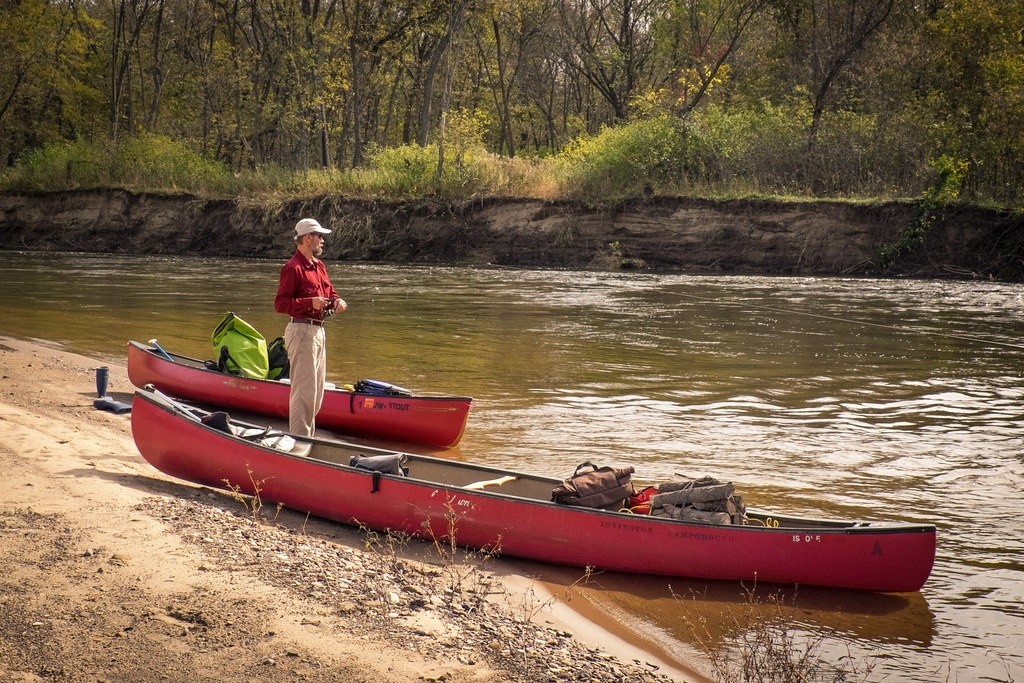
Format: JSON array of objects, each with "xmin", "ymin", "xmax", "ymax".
[{"xmin": 319, "ymin": 255, "xmax": 535, "ymax": 317}]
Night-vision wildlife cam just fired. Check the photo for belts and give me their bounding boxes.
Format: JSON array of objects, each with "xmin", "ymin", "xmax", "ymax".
[{"xmin": 290, "ymin": 318, "xmax": 325, "ymax": 327}]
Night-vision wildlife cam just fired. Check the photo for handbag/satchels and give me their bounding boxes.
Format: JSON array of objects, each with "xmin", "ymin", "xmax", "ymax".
[
  {"xmin": 349, "ymin": 453, "xmax": 409, "ymax": 493},
  {"xmin": 550, "ymin": 462, "xmax": 660, "ymax": 515}
]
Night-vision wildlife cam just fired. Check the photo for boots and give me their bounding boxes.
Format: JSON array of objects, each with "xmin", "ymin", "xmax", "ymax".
[{"xmin": 93, "ymin": 366, "xmax": 133, "ymax": 414}]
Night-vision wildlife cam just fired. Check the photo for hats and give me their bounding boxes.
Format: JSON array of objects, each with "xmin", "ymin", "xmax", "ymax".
[{"xmin": 294, "ymin": 219, "xmax": 331, "ymax": 240}]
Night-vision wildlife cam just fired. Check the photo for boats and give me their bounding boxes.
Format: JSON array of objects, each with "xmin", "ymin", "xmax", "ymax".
[
  {"xmin": 129, "ymin": 382, "xmax": 939, "ymax": 598},
  {"xmin": 125, "ymin": 337, "xmax": 473, "ymax": 449}
]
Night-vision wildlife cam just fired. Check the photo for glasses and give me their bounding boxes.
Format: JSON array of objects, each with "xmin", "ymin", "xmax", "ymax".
[{"xmin": 310, "ymin": 233, "xmax": 323, "ymax": 238}]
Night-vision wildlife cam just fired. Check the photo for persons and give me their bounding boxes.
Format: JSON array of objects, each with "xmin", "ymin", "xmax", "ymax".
[{"xmin": 273, "ymin": 217, "xmax": 349, "ymax": 439}]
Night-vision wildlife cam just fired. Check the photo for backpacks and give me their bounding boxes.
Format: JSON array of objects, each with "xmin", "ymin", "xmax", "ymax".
[
  {"xmin": 350, "ymin": 379, "xmax": 412, "ymax": 414},
  {"xmin": 204, "ymin": 310, "xmax": 290, "ymax": 381}
]
